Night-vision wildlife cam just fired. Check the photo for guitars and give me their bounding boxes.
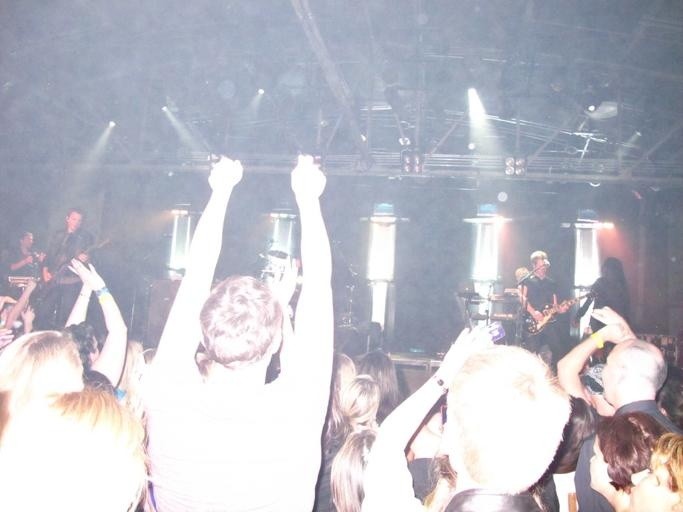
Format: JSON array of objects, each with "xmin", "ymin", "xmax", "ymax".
[
  {"xmin": 521, "ymin": 293, "xmax": 590, "ymax": 335},
  {"xmin": 36, "ymin": 236, "xmax": 109, "ymax": 300}
]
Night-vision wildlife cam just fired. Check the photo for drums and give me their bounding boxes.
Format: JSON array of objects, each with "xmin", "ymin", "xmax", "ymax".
[{"xmin": 335, "ymin": 321, "xmax": 381, "ymax": 355}]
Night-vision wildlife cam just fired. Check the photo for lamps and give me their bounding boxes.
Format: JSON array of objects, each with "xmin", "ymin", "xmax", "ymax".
[
  {"xmin": 398, "ymin": 145, "xmax": 425, "ymax": 176},
  {"xmin": 504, "ymin": 154, "xmax": 526, "ymax": 176}
]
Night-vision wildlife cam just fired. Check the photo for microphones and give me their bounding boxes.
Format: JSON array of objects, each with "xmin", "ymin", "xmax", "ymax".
[
  {"xmin": 543, "ymin": 259, "xmax": 551, "ymax": 268},
  {"xmin": 491, "ymin": 275, "xmax": 501, "ymax": 285},
  {"xmin": 33, "ymin": 251, "xmax": 39, "ymax": 258}
]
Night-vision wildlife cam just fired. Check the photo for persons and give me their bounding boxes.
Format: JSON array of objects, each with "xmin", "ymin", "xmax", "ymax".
[{"xmin": 1, "ymin": 151, "xmax": 683, "ymax": 511}]
